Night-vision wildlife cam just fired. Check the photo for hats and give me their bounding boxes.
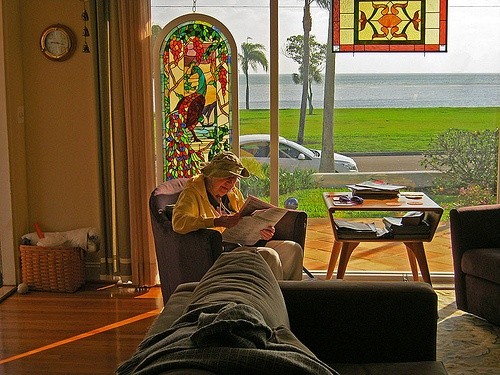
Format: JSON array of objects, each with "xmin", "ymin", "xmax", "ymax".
[{"xmin": 200, "ymin": 151, "xmax": 251, "ymax": 179}]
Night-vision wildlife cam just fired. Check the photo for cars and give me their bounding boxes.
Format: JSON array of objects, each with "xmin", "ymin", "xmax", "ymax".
[{"xmin": 239, "ymin": 134, "xmax": 358, "ymax": 173}]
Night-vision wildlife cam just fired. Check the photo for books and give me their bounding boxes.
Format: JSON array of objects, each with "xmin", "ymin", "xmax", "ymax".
[
  {"xmin": 345, "ymin": 178, "xmax": 406, "ymax": 199},
  {"xmin": 334, "ymin": 220, "xmax": 378, "ymax": 239},
  {"xmin": 383, "ymin": 216, "xmax": 432, "ymax": 239}
]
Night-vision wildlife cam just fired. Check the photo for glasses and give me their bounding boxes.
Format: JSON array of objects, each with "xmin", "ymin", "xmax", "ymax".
[{"xmin": 339, "ymin": 195, "xmax": 363, "ymax": 204}]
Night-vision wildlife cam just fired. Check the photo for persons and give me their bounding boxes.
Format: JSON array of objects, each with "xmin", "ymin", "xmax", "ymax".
[{"xmin": 171, "ymin": 151, "xmax": 304, "ymax": 281}]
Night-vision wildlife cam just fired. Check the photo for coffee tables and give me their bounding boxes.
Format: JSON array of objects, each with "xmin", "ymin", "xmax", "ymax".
[{"xmin": 323, "ymin": 191, "xmax": 443, "ymax": 321}]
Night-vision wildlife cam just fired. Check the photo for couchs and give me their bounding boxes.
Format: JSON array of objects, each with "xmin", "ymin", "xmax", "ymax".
[
  {"xmin": 121, "ymin": 246, "xmax": 448, "ymax": 375},
  {"xmin": 149, "ymin": 178, "xmax": 307, "ymax": 307},
  {"xmin": 448, "ymin": 204, "xmax": 500, "ymax": 328}
]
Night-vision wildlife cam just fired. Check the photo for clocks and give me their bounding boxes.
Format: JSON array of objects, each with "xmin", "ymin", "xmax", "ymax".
[{"xmin": 40, "ymin": 24, "xmax": 74, "ymax": 62}]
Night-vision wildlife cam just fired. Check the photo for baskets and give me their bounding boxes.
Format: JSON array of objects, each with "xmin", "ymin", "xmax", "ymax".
[{"xmin": 20, "ymin": 244, "xmax": 87, "ymax": 293}]
